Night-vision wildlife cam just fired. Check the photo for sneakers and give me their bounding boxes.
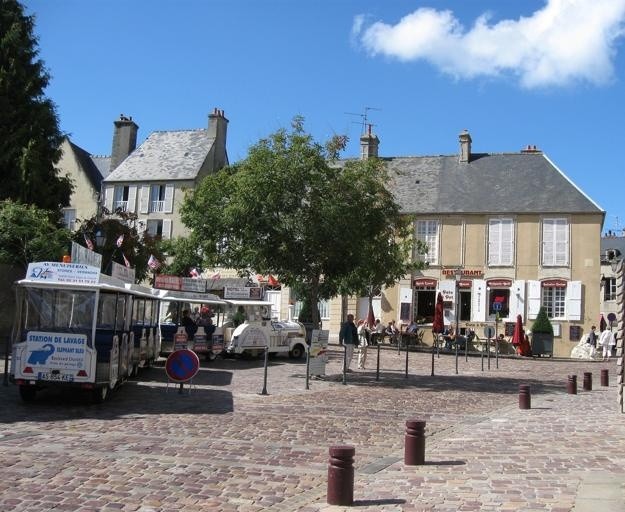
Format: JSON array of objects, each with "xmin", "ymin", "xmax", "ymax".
[{"xmin": 346, "ymin": 369, "xmax": 352, "ymax": 372}]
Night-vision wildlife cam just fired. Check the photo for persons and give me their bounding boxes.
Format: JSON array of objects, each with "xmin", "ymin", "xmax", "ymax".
[
  {"xmin": 168, "ymin": 304, "xmax": 249, "ymax": 356},
  {"xmin": 356, "ymin": 320, "xmax": 373, "ymax": 370},
  {"xmin": 353, "ymin": 317, "xmax": 418, "ymax": 346},
  {"xmin": 585, "ymin": 326, "xmax": 598, "ymax": 360},
  {"xmin": 599, "ymin": 327, "xmax": 616, "ymax": 362},
  {"xmin": 339, "ymin": 313, "xmax": 361, "ymax": 373}
]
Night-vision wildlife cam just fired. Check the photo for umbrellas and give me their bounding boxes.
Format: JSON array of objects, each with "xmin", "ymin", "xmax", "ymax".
[
  {"xmin": 432, "ymin": 292, "xmax": 445, "ymax": 358},
  {"xmin": 511, "ymin": 314, "xmax": 525, "ymax": 357},
  {"xmin": 366, "ymin": 296, "xmax": 376, "ymax": 331}
]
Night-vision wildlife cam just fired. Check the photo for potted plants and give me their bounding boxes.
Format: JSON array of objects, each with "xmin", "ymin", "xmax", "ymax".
[{"xmin": 531, "ymin": 307, "xmax": 553, "ymax": 357}]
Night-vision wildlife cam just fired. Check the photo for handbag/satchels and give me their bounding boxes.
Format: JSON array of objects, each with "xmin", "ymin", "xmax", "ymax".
[{"xmin": 586, "ymin": 337, "xmax": 590, "ymax": 342}]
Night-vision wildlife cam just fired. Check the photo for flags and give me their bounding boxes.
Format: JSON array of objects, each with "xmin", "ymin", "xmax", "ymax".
[
  {"xmin": 148, "ymin": 253, "xmax": 162, "ymax": 270},
  {"xmin": 83, "ymin": 233, "xmax": 94, "ymax": 250},
  {"xmin": 123, "ymin": 254, "xmax": 131, "ymax": 268},
  {"xmin": 116, "ymin": 234, "xmax": 125, "ymax": 247}
]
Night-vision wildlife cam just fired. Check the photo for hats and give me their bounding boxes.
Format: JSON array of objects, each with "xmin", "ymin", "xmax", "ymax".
[{"xmin": 358, "ymin": 319, "xmax": 364, "ymax": 325}]
